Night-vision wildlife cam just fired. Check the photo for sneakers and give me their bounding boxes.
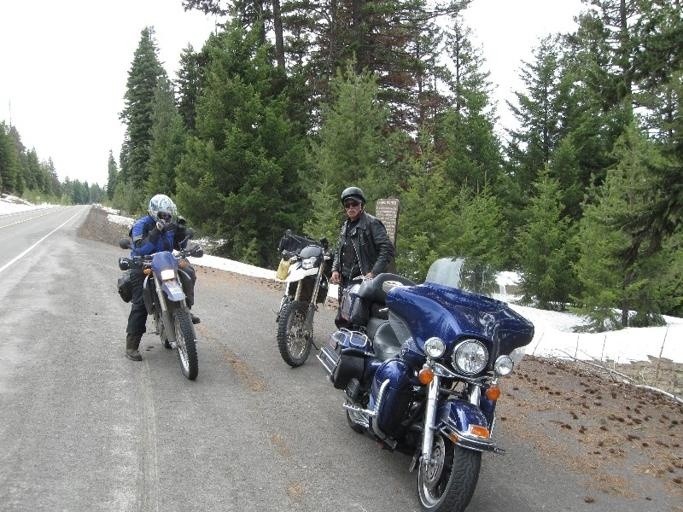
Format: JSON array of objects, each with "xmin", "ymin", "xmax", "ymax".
[
  {"xmin": 123, "ymin": 346, "xmax": 143, "ymax": 361},
  {"xmin": 189, "ymin": 310, "xmax": 201, "ymax": 325}
]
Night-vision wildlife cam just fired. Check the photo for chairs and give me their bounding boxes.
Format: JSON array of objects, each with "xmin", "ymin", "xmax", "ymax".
[{"xmin": 357, "ymin": 273, "xmax": 418, "ymax": 339}]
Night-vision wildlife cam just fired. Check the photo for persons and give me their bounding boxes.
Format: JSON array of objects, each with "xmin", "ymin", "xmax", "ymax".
[
  {"xmin": 332, "ymin": 187, "xmax": 395, "ymax": 326},
  {"xmin": 125, "ymin": 193, "xmax": 200, "ymax": 361}
]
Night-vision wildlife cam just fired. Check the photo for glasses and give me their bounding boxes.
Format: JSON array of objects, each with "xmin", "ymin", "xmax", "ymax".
[{"xmin": 343, "ymin": 201, "xmax": 362, "ymax": 209}]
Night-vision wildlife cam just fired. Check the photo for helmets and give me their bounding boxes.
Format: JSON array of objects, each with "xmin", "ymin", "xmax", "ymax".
[
  {"xmin": 339, "ymin": 185, "xmax": 367, "ymax": 207},
  {"xmin": 146, "ymin": 191, "xmax": 179, "ymax": 229}
]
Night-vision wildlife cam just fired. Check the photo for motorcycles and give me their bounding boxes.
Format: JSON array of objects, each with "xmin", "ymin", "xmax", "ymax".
[
  {"xmin": 277, "ymin": 230, "xmax": 332, "ymax": 368},
  {"xmin": 315, "ymin": 272, "xmax": 535, "ymax": 512},
  {"xmin": 116, "ymin": 226, "xmax": 204, "ymax": 381}
]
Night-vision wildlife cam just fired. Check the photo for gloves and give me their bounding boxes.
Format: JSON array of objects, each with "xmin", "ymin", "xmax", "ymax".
[
  {"xmin": 154, "ymin": 218, "xmax": 167, "ymax": 232},
  {"xmin": 175, "ymin": 214, "xmax": 187, "ymax": 228}
]
